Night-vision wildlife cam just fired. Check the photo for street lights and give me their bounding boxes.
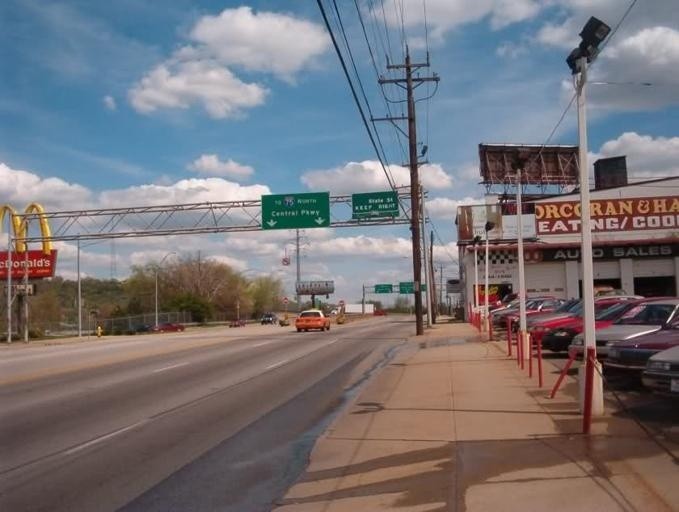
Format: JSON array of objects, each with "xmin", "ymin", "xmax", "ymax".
[
  {"xmin": 155, "ymin": 251, "xmax": 177, "ymax": 326},
  {"xmin": 565, "ymin": 20, "xmax": 612, "ymax": 417},
  {"xmin": 483, "ymin": 219, "xmax": 497, "ymax": 334},
  {"xmin": 76, "ymin": 232, "xmax": 115, "ymax": 337},
  {"xmin": 471, "ymin": 233, "xmax": 483, "ymax": 313}
]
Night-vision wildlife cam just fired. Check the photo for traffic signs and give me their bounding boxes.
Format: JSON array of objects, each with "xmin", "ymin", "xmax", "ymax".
[
  {"xmin": 420, "ymin": 284, "xmax": 427, "ymax": 291},
  {"xmin": 374, "ymin": 284, "xmax": 393, "ymax": 293},
  {"xmin": 399, "ymin": 282, "xmax": 415, "ymax": 294},
  {"xmin": 351, "ymin": 191, "xmax": 400, "ymax": 220},
  {"xmin": 260, "ymin": 192, "xmax": 332, "ymax": 231}
]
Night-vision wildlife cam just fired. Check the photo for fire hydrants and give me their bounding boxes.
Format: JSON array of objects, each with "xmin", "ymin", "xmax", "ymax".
[{"xmin": 95, "ymin": 325, "xmax": 105, "ymax": 337}]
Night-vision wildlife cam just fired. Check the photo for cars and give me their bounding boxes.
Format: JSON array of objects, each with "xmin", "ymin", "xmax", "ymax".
[
  {"xmin": 261, "ymin": 313, "xmax": 279, "ymax": 325},
  {"xmin": 295, "ymin": 310, "xmax": 331, "ymax": 331},
  {"xmin": 472, "ymin": 287, "xmax": 679, "ymax": 405},
  {"xmin": 131, "ymin": 322, "xmax": 151, "ymax": 332},
  {"xmin": 150, "ymin": 322, "xmax": 185, "ymax": 334},
  {"xmin": 374, "ymin": 310, "xmax": 386, "ymax": 317},
  {"xmin": 229, "ymin": 320, "xmax": 247, "ymax": 328}
]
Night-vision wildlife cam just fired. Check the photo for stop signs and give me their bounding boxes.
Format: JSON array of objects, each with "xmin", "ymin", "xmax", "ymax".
[
  {"xmin": 338, "ymin": 300, "xmax": 344, "ymax": 306},
  {"xmin": 282, "ymin": 298, "xmax": 290, "ymax": 304}
]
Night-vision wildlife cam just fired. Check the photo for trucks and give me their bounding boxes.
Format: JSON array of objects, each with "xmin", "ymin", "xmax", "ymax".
[{"xmin": 331, "ymin": 303, "xmax": 376, "ymax": 315}]
